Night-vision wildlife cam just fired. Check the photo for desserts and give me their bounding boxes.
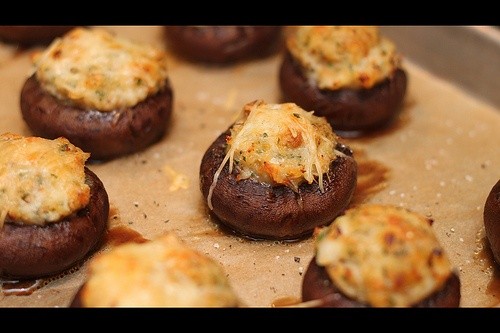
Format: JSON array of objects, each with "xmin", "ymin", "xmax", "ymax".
[
  {"xmin": 0, "ymin": 132, "xmax": 110, "ymax": 281},
  {"xmin": 67, "ymin": 240, "xmax": 240, "ymax": 308},
  {"xmin": 300, "ymin": 180, "xmax": 500, "ymax": 308},
  {"xmin": 0, "ymin": 25, "xmax": 277, "ymax": 162},
  {"xmin": 199, "ymin": 99, "xmax": 357, "ymax": 241},
  {"xmin": 278, "ymin": 27, "xmax": 408, "ymax": 134}
]
